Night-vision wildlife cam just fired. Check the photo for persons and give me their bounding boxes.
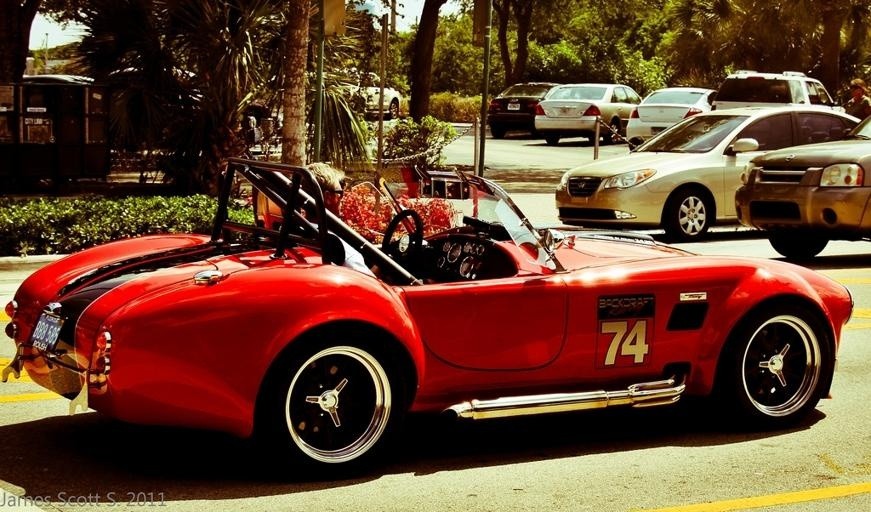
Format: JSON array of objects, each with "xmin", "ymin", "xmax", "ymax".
[
  {"xmin": 846, "ymin": 78, "xmax": 871, "ymax": 120},
  {"xmin": 300, "ymin": 161, "xmax": 383, "ymax": 279}
]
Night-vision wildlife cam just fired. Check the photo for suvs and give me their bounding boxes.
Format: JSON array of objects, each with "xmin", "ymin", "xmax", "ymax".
[
  {"xmin": 732, "ymin": 112, "xmax": 871, "ymax": 257},
  {"xmin": 710, "ymin": 69, "xmax": 845, "ymax": 113}
]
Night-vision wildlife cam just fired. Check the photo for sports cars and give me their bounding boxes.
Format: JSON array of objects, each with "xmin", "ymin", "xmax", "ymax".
[{"xmin": 5, "ymin": 160, "xmax": 854, "ymax": 478}]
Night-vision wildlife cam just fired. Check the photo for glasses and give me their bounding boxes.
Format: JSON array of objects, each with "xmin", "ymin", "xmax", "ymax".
[{"xmin": 329, "ymin": 190, "xmax": 343, "ymax": 199}]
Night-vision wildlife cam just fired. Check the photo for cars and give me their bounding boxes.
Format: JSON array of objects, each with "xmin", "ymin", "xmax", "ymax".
[
  {"xmin": 556, "ymin": 106, "xmax": 864, "ymax": 242},
  {"xmin": 24, "ymin": 64, "xmax": 196, "ymax": 89},
  {"xmin": 626, "ymin": 87, "xmax": 717, "ymax": 151},
  {"xmin": 533, "ymin": 84, "xmax": 642, "ymax": 146},
  {"xmin": 353, "ymin": 72, "xmax": 402, "ymax": 119},
  {"xmin": 487, "ymin": 82, "xmax": 563, "ymax": 138}
]
